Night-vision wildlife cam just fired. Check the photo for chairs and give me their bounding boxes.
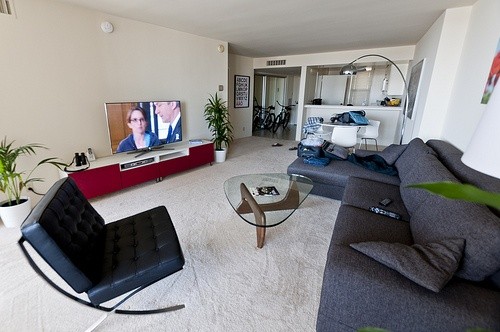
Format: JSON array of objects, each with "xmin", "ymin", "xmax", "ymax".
[
  {"xmin": 331, "ymin": 126, "xmax": 357, "ymax": 148},
  {"xmin": 305, "ymin": 117, "xmax": 328, "ymax": 140},
  {"xmin": 357, "ymin": 120, "xmax": 380, "ymax": 151},
  {"xmin": 17, "ymin": 177, "xmax": 185, "ymax": 332}
]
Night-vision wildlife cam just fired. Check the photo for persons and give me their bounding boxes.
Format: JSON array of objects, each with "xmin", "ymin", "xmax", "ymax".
[
  {"xmin": 154, "ymin": 102, "xmax": 181, "ymax": 143},
  {"xmin": 117, "ymin": 107, "xmax": 162, "ymax": 151}
]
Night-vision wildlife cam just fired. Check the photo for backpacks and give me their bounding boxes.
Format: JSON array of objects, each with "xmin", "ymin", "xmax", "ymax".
[{"xmin": 322, "ymin": 142, "xmax": 349, "ymax": 161}]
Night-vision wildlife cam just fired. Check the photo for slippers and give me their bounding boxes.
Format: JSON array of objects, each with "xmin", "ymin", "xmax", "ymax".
[
  {"xmin": 272, "ymin": 142, "xmax": 284, "ymax": 147},
  {"xmin": 289, "ymin": 147, "xmax": 298, "ymax": 150}
]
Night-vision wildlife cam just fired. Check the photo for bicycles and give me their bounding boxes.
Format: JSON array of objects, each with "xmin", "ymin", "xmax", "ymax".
[
  {"xmin": 272, "ymin": 101, "xmax": 296, "ymax": 133},
  {"xmin": 253, "ymin": 96, "xmax": 275, "ymax": 132}
]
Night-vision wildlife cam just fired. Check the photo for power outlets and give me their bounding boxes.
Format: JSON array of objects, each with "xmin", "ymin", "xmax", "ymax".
[{"xmin": 218, "ymin": 85, "xmax": 223, "ymax": 90}]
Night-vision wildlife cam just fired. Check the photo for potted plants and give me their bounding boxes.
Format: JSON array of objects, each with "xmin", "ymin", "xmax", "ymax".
[
  {"xmin": 202, "ymin": 92, "xmax": 239, "ymax": 163},
  {"xmin": 0, "ymin": 134, "xmax": 70, "ymax": 228}
]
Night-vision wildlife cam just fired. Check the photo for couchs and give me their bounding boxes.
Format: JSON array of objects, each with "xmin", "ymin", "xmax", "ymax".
[
  {"xmin": 316, "ymin": 152, "xmax": 500, "ymax": 331},
  {"xmin": 288, "ymin": 138, "xmax": 464, "ymax": 202}
]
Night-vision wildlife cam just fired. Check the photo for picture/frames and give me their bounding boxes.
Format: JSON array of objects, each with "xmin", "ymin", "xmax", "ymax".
[
  {"xmin": 233, "ymin": 75, "xmax": 250, "ymax": 109},
  {"xmin": 402, "ymin": 59, "xmax": 425, "ymax": 119}
]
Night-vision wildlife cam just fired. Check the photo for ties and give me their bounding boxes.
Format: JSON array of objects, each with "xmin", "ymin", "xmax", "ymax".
[{"xmin": 166, "ymin": 125, "xmax": 172, "ymax": 144}]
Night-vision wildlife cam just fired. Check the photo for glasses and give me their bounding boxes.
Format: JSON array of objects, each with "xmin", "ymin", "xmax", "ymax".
[{"xmin": 130, "ymin": 118, "xmax": 145, "ymax": 122}]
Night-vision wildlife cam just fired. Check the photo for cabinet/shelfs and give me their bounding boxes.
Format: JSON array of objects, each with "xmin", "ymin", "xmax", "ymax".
[
  {"xmin": 382, "ymin": 64, "xmax": 409, "ymax": 96},
  {"xmin": 59, "ymin": 138, "xmax": 215, "ymax": 199}
]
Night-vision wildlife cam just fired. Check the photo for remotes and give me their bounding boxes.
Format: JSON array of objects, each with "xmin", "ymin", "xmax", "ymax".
[
  {"xmin": 379, "ymin": 198, "xmax": 393, "ymax": 207},
  {"xmin": 368, "ymin": 206, "xmax": 400, "ymax": 219}
]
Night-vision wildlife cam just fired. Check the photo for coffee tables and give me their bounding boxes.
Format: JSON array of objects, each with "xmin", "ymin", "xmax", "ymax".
[{"xmin": 223, "ymin": 173, "xmax": 314, "ymax": 249}]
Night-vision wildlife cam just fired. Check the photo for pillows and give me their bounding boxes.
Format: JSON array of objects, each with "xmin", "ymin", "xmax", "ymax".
[
  {"xmin": 378, "ymin": 144, "xmax": 408, "ymax": 165},
  {"xmin": 394, "ymin": 137, "xmax": 437, "ymax": 182},
  {"xmin": 349, "ymin": 239, "xmax": 466, "ymax": 294},
  {"xmin": 399, "ymin": 154, "xmax": 461, "ymax": 215},
  {"xmin": 409, "ymin": 181, "xmax": 500, "ymax": 283}
]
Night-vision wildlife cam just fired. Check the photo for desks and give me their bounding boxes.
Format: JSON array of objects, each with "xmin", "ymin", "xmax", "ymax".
[{"xmin": 320, "ymin": 121, "xmax": 370, "ymax": 132}]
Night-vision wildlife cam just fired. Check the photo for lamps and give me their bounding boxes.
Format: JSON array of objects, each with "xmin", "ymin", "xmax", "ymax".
[{"xmin": 339, "ymin": 55, "xmax": 409, "ymax": 143}]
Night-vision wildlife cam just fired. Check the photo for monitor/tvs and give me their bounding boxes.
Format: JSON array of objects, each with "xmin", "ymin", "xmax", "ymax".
[{"xmin": 104, "ymin": 100, "xmax": 182, "ymax": 154}]
